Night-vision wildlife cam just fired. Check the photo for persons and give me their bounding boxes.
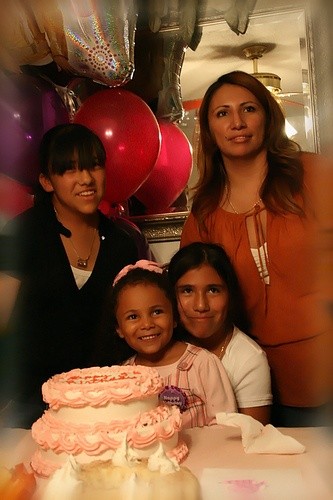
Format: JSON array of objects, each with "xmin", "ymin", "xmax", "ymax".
[
  {"xmin": 111, "ymin": 259, "xmax": 237, "ymax": 425},
  {"xmin": 169, "ymin": 242, "xmax": 274, "ymax": 429},
  {"xmin": 0, "ymin": 122, "xmax": 156, "ymax": 431},
  {"xmin": 180, "ymin": 71, "xmax": 333, "ymax": 428}
]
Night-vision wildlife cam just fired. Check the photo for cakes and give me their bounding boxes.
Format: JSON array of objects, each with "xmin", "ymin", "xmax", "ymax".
[{"xmin": 31, "ymin": 364, "xmax": 188, "ymax": 479}]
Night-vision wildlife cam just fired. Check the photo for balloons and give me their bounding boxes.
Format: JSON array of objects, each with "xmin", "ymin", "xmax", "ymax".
[
  {"xmin": 0, "ymin": 0, "xmax": 198, "ymax": 126},
  {"xmin": 133, "ymin": 118, "xmax": 194, "ymax": 212},
  {"xmin": 74, "ymin": 88, "xmax": 162, "ymax": 210}
]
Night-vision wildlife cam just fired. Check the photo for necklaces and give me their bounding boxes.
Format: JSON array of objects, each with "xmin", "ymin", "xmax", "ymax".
[
  {"xmin": 218, "ymin": 334, "xmax": 228, "ymax": 359},
  {"xmin": 54, "ymin": 207, "xmax": 98, "ymax": 269},
  {"xmin": 225, "ymin": 188, "xmax": 263, "ymax": 214}
]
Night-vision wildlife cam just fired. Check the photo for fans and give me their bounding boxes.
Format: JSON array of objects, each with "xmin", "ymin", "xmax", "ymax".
[{"xmin": 242, "ymin": 45, "xmax": 302, "ymax": 97}]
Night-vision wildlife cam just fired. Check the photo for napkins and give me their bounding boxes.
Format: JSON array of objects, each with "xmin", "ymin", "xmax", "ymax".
[{"xmin": 216, "ymin": 412, "xmax": 306, "ymax": 454}]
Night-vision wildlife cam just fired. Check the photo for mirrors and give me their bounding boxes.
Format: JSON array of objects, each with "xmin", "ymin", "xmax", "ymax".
[{"xmin": 112, "ymin": 0, "xmax": 333, "ymax": 244}]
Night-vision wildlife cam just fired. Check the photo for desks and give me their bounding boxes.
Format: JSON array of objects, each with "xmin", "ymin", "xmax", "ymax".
[{"xmin": 0, "ymin": 426, "xmax": 333, "ymax": 500}]
